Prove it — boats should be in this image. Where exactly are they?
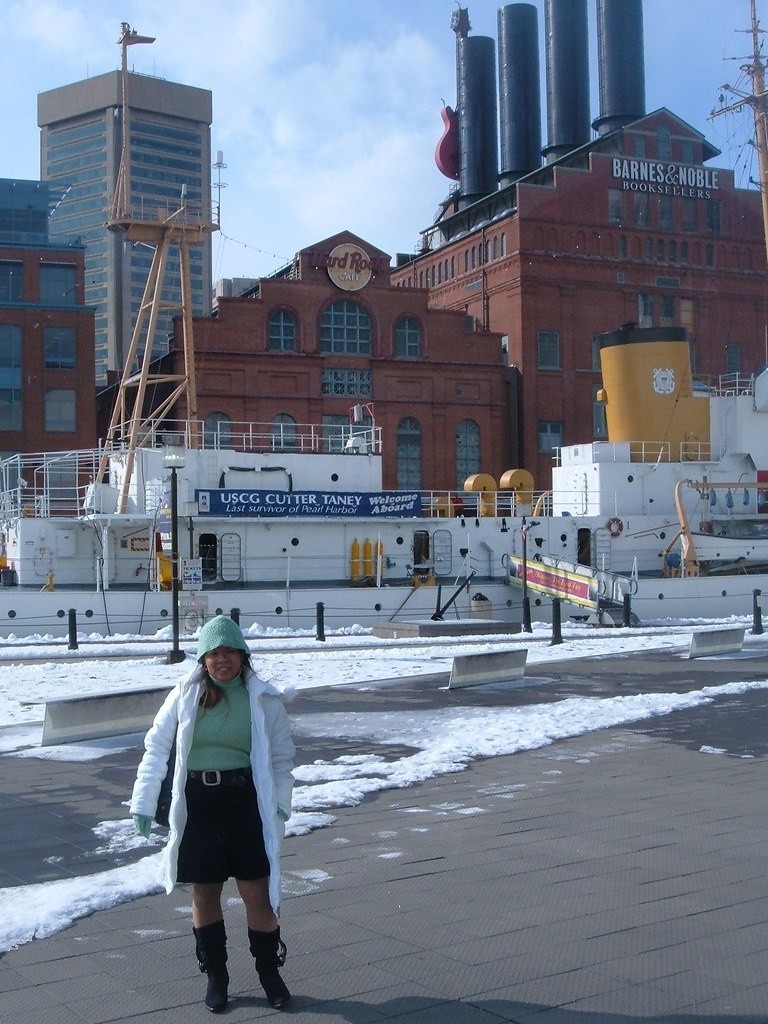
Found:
[689,530,768,567]
[0,19,768,646]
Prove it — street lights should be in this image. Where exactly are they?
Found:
[161,443,186,665]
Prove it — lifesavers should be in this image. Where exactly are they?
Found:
[607,518,623,536]
[184,611,203,631]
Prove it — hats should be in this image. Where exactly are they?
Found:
[196,615,251,664]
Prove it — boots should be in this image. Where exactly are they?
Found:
[247,924,291,1008]
[192,919,230,1012]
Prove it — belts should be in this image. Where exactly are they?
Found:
[188,768,252,785]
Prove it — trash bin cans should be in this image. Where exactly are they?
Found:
[178,595,210,635]
[470,592,493,620]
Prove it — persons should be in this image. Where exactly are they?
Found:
[129,615,295,1012]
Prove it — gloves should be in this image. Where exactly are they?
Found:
[133,814,152,840]
